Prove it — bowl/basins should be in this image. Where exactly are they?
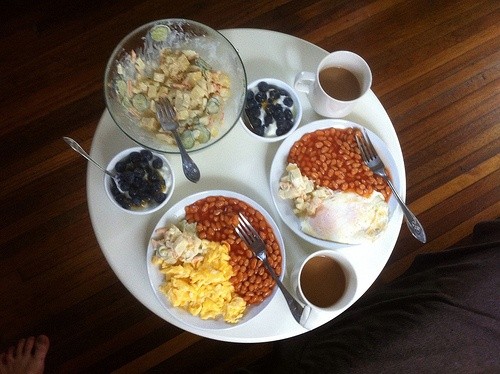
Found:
[240,78,302,142]
[103,17,247,158]
[104,147,176,214]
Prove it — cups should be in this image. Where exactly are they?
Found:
[289,249,358,327]
[293,50,373,119]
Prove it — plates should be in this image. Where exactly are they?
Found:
[269,118,401,250]
[145,188,287,329]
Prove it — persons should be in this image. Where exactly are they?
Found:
[0,335,48,374]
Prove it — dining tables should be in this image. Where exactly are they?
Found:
[86,29,407,344]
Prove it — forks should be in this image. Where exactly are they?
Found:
[233,211,310,327]
[156,96,202,184]
[356,133,428,245]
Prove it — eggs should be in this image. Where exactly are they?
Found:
[297,191,389,246]
